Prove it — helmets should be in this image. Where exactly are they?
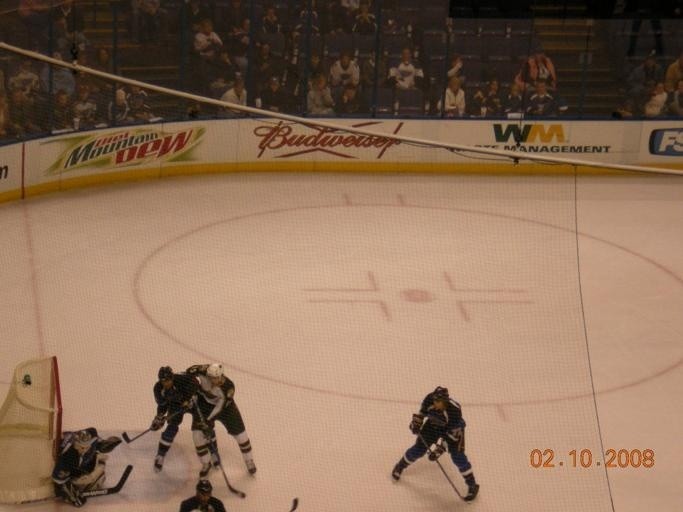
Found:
[205,363,223,377]
[73,430,92,449]
[194,479,212,493]
[430,386,449,402]
[157,366,174,380]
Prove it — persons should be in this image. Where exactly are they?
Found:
[612,52,683,118]
[132,0,559,121]
[392,386,480,500]
[0,0,155,139]
[152,367,220,468]
[52,428,123,506]
[180,479,226,512]
[184,363,256,476]
[625,2,664,57]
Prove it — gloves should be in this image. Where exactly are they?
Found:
[428,444,446,461]
[150,415,165,431]
[409,414,424,435]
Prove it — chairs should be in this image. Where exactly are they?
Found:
[0,0,682,140]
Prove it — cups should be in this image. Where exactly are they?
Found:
[392,100,399,112]
[480,104,487,118]
[423,99,430,112]
[72,118,80,130]
[253,96,261,108]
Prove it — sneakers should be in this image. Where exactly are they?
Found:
[209,451,222,467]
[154,456,163,471]
[463,484,479,501]
[246,460,256,474]
[392,464,402,479]
[198,462,211,477]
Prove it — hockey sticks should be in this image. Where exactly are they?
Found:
[414,427,475,501]
[123,411,183,444]
[195,406,246,498]
[291,497,299,511]
[16,465,132,504]
